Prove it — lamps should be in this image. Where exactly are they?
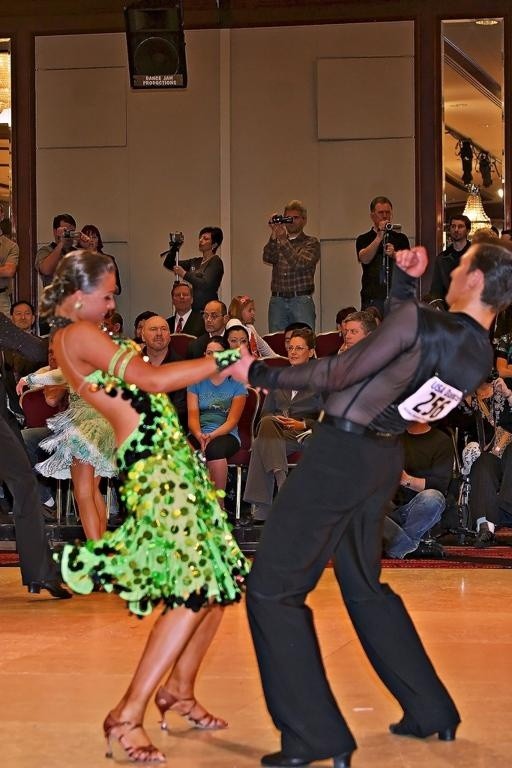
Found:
[459,143,491,241]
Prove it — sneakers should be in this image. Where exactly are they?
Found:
[404,523,494,560]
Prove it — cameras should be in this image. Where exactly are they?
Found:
[169,231,184,243]
[64,229,81,239]
[385,222,402,231]
[272,215,293,224]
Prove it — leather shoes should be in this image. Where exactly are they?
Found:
[261,752,353,768]
[28,580,73,600]
[388,723,458,741]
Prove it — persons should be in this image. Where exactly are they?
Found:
[43,250,242,764]
[3,197,510,597]
[210,242,511,768]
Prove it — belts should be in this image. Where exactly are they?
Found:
[271,289,311,298]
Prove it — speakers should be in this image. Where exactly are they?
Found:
[123,5,188,89]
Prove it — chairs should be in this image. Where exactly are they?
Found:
[20,327,487,528]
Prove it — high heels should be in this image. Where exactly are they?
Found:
[155,684,229,731]
[103,711,167,764]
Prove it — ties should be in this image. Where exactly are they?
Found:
[175,317,184,335]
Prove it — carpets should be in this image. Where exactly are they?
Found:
[0,530,512,570]
